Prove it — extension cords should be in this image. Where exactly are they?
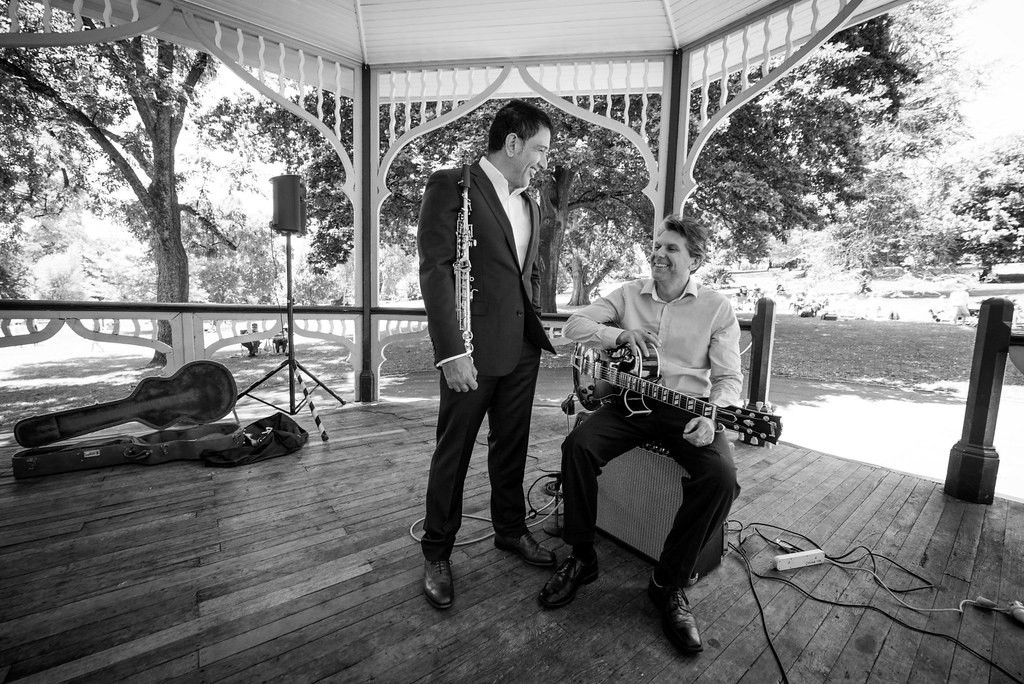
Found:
[773,549,825,570]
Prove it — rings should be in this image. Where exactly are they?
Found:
[702,440,705,445]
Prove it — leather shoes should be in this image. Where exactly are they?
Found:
[494,532,557,566]
[649,570,703,653]
[538,550,599,607]
[423,558,454,608]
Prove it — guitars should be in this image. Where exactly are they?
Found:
[569,321,784,450]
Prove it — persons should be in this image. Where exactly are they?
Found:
[419,100,558,602]
[536,216,744,653]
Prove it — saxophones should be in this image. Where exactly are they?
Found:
[451,149,478,362]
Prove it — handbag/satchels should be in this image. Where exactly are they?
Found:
[200,412,309,468]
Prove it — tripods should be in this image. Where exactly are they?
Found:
[235,233,347,442]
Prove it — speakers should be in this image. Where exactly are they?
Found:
[268,175,306,235]
[573,411,724,586]
